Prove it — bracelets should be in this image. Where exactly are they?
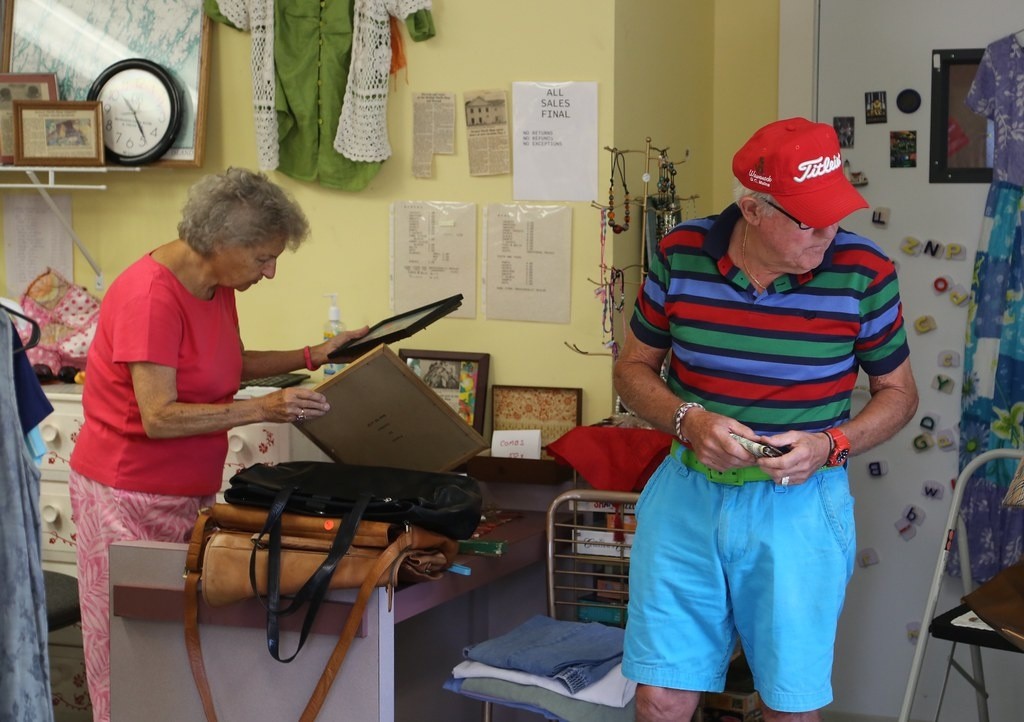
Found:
[820,431,832,467]
[304,346,320,371]
[672,402,705,443]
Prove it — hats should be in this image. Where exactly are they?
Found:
[733,117,870,229]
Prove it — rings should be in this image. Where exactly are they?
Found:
[782,476,789,485]
[297,408,306,421]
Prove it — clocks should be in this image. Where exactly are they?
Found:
[87,59,182,165]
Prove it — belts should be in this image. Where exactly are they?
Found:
[670,440,773,485]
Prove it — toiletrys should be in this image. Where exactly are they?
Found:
[319,293,347,375]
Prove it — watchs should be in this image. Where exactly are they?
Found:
[825,428,851,467]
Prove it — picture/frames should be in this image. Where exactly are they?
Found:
[329,292,464,358]
[294,343,489,472]
[0,0,213,167]
[0,71,60,165]
[490,384,582,459]
[397,349,490,474]
[13,98,105,166]
[928,46,996,183]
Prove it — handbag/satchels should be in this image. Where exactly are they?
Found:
[184,457,487,722]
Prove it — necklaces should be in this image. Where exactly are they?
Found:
[742,223,766,289]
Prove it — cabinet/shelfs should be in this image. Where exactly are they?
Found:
[25,384,86,564]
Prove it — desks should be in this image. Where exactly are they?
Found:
[107,508,583,722]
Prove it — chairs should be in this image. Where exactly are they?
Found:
[482,489,704,722]
[900,449,1024,722]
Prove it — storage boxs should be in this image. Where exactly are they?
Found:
[572,528,636,557]
[597,579,629,598]
[568,501,636,514]
[605,513,636,530]
[704,687,762,722]
[576,593,628,624]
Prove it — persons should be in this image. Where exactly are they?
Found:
[613,117,919,722]
[67,168,369,722]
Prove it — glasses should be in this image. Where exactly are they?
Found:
[761,197,812,230]
